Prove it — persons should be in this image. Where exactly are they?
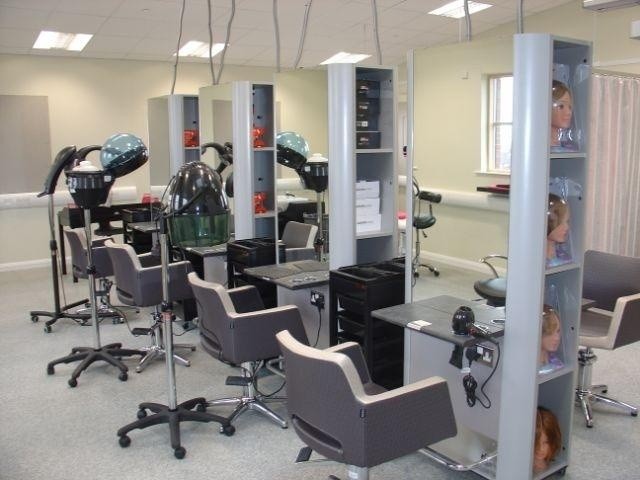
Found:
[551,80,572,146]
[541,303,562,367]
[547,192,571,260]
[533,407,562,472]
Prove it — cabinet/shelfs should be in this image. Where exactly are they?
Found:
[496,32,594,480]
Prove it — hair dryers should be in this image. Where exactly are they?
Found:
[447,306,499,369]
